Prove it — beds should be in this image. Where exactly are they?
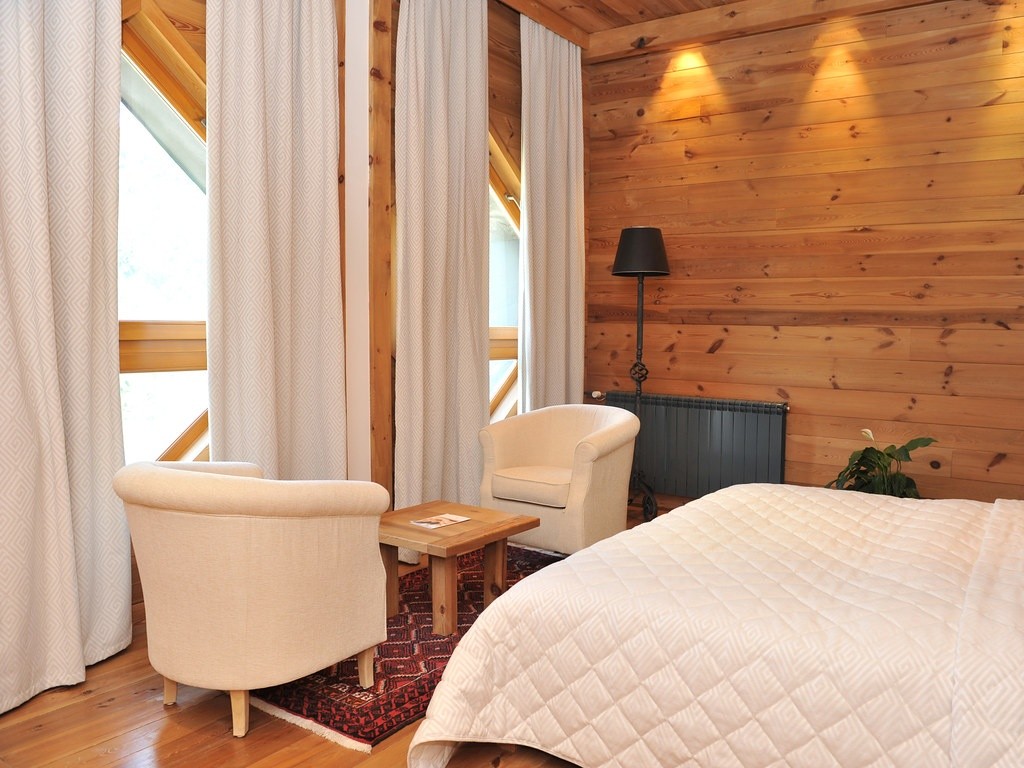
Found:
[406,483,1024,768]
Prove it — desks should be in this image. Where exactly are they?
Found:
[380,500,540,637]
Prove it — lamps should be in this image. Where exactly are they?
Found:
[609,227,670,514]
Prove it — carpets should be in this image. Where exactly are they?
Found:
[222,543,566,754]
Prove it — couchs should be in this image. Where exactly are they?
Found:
[113,459,391,739]
[479,403,642,558]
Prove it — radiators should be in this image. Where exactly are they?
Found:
[592,390,792,499]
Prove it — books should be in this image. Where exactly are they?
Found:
[410,513,471,529]
